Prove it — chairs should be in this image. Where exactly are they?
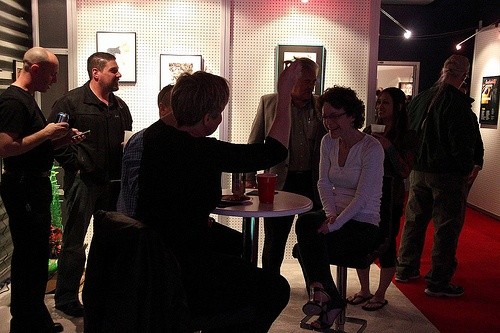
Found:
[92,209,198,333]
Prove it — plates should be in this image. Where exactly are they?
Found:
[221,194,253,204]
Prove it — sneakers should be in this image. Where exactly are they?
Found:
[425,284,465,297]
[395,270,421,282]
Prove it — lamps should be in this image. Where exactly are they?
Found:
[456,23,494,50]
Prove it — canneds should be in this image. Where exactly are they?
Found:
[55,112,69,123]
[232,173,246,195]
[246,172,257,188]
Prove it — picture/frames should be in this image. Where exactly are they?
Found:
[95,31,136,84]
[159,53,203,91]
[277,44,326,97]
[12,60,23,82]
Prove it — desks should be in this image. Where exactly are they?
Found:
[212,188,313,270]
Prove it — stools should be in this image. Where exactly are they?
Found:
[293,242,380,332]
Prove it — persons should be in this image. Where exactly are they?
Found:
[348,87,418,309]
[295,86,385,329]
[244,58,321,273]
[47,51,132,318]
[0,47,86,333]
[394,55,485,296]
[116,60,302,333]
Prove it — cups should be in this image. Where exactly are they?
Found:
[231,172,246,201]
[257,173,278,206]
[371,123,385,138]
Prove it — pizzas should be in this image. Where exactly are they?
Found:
[221,195,241,200]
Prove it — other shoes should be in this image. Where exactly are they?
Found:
[55,302,84,317]
[53,322,63,331]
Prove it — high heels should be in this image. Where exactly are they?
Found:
[311,303,346,328]
[302,287,332,316]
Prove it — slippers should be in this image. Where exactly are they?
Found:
[346,293,373,304]
[362,298,388,311]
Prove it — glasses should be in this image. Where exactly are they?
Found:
[319,112,345,121]
